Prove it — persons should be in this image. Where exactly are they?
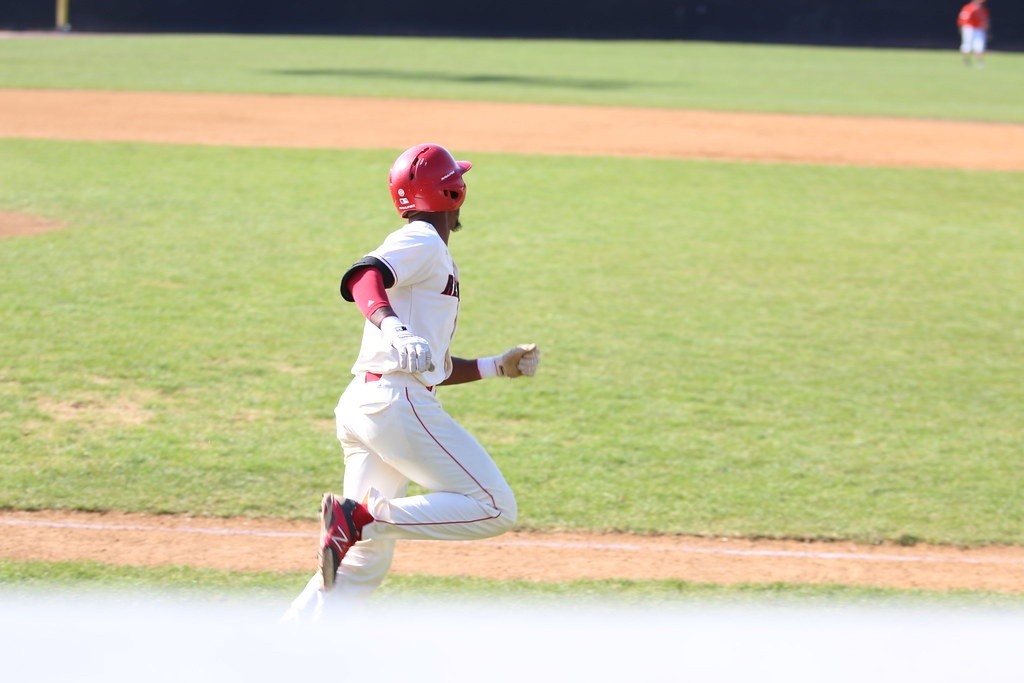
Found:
[957,0,990,63]
[277,143,539,618]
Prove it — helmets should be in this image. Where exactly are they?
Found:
[388,144,473,219]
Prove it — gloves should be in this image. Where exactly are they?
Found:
[477,343,539,380]
[380,315,436,374]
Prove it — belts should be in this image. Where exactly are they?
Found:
[365,372,433,393]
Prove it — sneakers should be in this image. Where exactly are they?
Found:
[320,492,363,593]
